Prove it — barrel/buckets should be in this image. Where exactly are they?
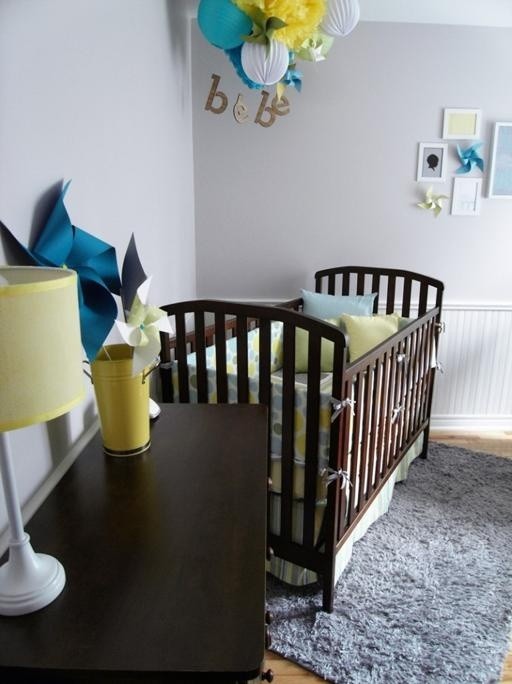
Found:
[82,343,160,457]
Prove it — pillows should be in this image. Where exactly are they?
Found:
[288,287,402,375]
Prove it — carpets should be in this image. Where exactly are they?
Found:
[267,439,512,683]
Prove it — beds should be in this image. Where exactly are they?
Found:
[156,265,445,614]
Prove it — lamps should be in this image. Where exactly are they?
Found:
[0,266,98,617]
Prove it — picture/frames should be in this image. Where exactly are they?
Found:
[414,106,511,217]
